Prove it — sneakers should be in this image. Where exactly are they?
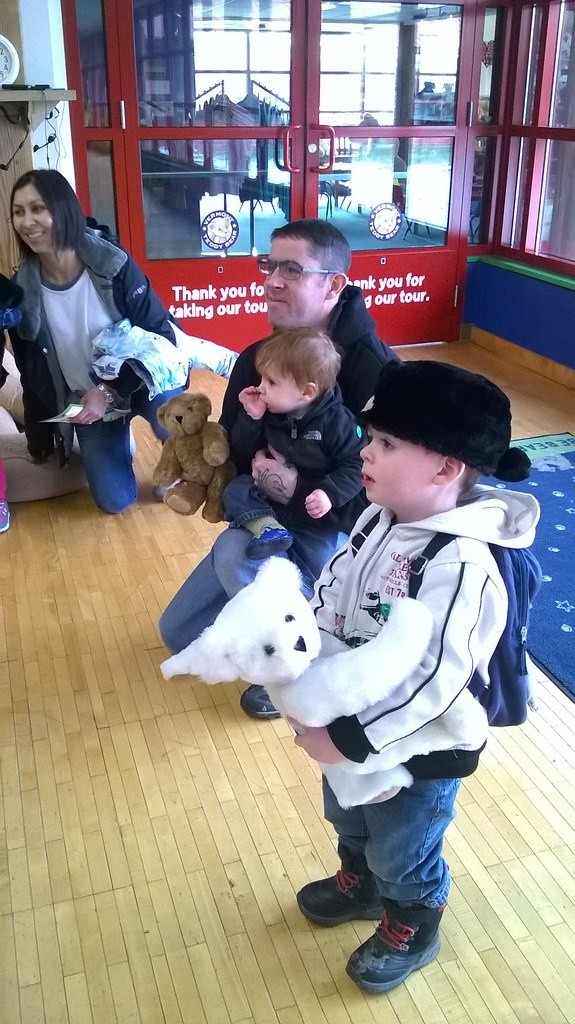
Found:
[0,499,10,533]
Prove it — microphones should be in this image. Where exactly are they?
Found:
[0,140,26,171]
[34,135,57,152]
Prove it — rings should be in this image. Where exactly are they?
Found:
[88,421,92,424]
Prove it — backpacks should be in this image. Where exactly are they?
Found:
[349,507,542,728]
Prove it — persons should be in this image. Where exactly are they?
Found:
[0,169,184,533]
[224,328,367,582]
[291,360,539,994]
[160,218,400,721]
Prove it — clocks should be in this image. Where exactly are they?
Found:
[0,34,20,91]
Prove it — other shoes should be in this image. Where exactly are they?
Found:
[128,421,136,461]
[152,476,181,501]
[245,524,294,560]
[240,684,284,719]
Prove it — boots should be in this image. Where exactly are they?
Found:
[297,843,384,926]
[346,896,447,993]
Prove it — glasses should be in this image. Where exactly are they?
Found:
[256,257,340,280]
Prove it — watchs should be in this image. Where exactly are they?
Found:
[97,384,115,404]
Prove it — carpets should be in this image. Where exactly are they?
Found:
[476,432,575,700]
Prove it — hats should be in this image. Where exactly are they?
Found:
[356,359,531,482]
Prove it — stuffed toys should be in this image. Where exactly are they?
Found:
[153,393,235,523]
[163,556,486,809]
